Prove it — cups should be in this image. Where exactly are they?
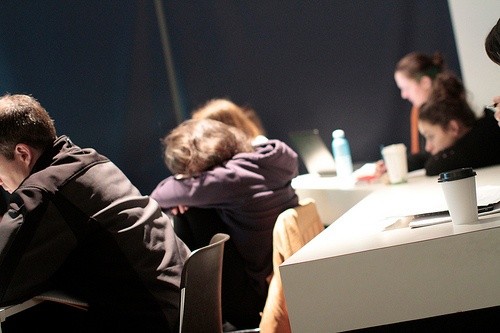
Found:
[438,167,479,226]
[381,143,408,184]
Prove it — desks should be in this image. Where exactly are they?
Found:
[279,163,500,333]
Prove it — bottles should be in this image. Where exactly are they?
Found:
[331,130,354,178]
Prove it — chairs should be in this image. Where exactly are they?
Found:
[178,233,230,332]
[258,201,326,333]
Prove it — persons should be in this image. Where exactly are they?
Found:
[150,98,299,333]
[0,94,191,333]
[376,18,500,176]
